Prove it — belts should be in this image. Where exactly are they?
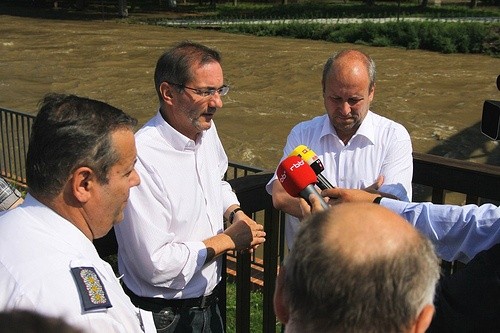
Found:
[125,286,221,310]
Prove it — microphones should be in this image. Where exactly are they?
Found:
[276,154,330,210]
[288,144,337,198]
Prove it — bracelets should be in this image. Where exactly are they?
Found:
[229,208,242,225]
[373,197,382,204]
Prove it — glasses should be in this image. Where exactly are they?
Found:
[163,79,232,98]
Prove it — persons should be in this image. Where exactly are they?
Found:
[0,92,158,333]
[320,188,500,264]
[273,201,440,333]
[266,49,413,254]
[113,42,267,333]
